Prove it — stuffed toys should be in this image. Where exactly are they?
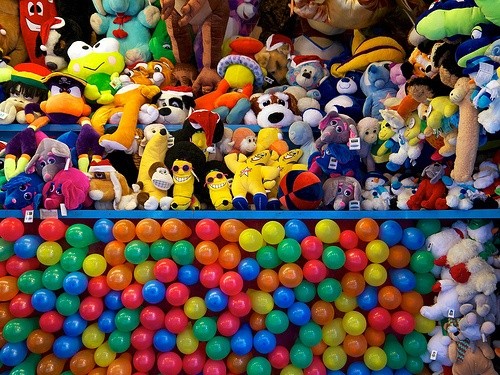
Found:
[420,220,500,375]
[0,0,500,210]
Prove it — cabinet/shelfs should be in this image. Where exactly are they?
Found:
[0,123,500,220]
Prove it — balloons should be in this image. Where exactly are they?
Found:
[0,216,441,375]
